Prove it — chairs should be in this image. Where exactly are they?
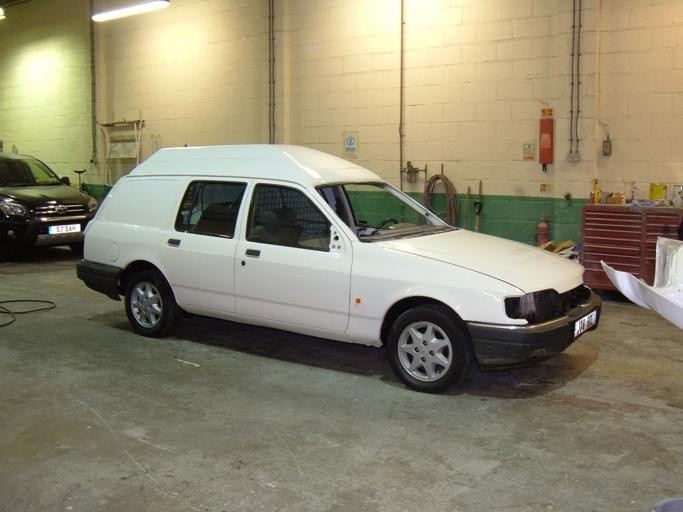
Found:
[255,208,303,244]
[191,204,234,237]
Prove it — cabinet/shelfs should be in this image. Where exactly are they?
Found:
[580,205,682,291]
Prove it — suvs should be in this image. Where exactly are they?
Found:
[0,152,100,258]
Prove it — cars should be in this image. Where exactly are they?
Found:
[73,143,601,392]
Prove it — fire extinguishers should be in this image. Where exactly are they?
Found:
[537,217,548,246]
